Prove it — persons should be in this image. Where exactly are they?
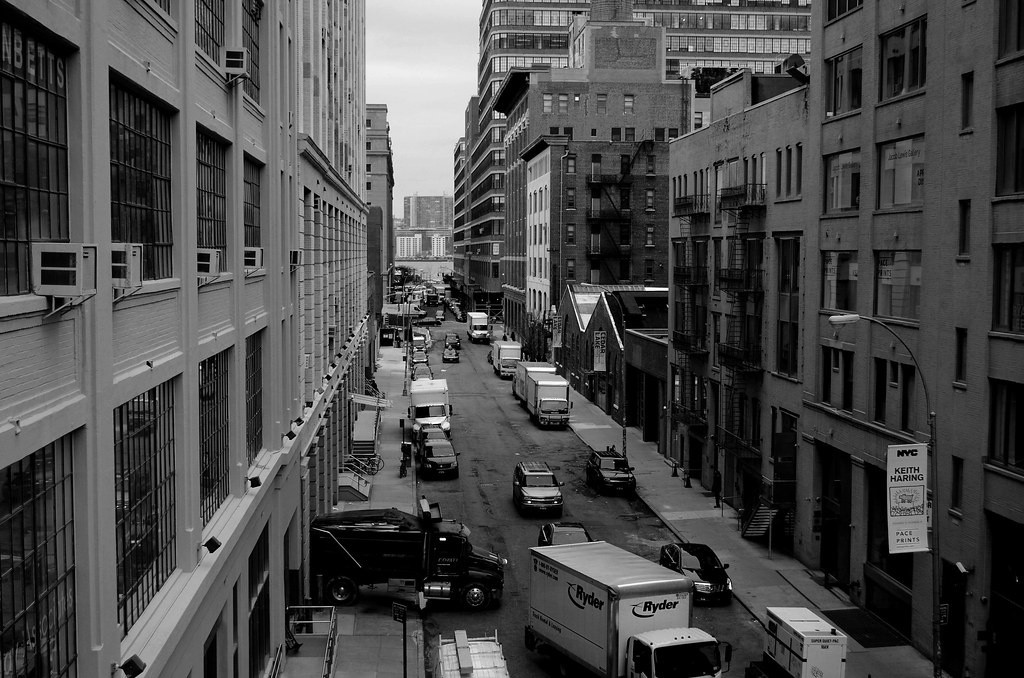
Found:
[502,334,507,341]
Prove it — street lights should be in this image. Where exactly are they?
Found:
[831,313,943,678]
[402,268,424,353]
[580,283,626,457]
[403,279,440,395]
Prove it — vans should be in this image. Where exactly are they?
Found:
[427,294,439,307]
[381,303,427,318]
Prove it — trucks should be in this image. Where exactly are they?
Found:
[494,340,522,378]
[523,541,733,678]
[516,361,558,409]
[467,312,491,344]
[309,502,507,609]
[407,378,453,440]
[433,285,446,300]
[525,372,573,428]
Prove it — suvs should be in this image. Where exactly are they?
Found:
[513,460,565,515]
[587,449,637,494]
[417,424,453,456]
[538,521,591,547]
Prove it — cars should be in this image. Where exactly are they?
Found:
[443,333,462,349]
[411,336,434,380]
[421,439,460,480]
[436,310,445,321]
[487,350,494,364]
[412,317,442,327]
[442,298,467,322]
[660,543,733,603]
[441,345,460,363]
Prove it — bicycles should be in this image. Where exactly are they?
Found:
[346,454,384,475]
[399,459,408,478]
